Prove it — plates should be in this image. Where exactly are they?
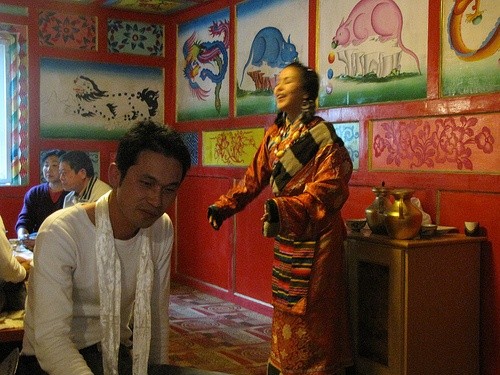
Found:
[435,225,456,234]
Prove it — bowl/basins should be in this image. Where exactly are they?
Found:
[345,218,367,233]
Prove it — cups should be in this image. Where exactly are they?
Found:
[419,224,437,239]
[463,222,479,237]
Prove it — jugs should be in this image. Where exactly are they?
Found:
[383,190,422,240]
[365,186,393,235]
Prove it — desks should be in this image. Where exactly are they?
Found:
[9,239,35,293]
[342,223,488,375]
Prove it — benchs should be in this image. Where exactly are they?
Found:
[0,307,25,343]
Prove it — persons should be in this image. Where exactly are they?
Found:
[0,215,31,362]
[15,149,114,252]
[207,62,353,375]
[18,119,226,375]
